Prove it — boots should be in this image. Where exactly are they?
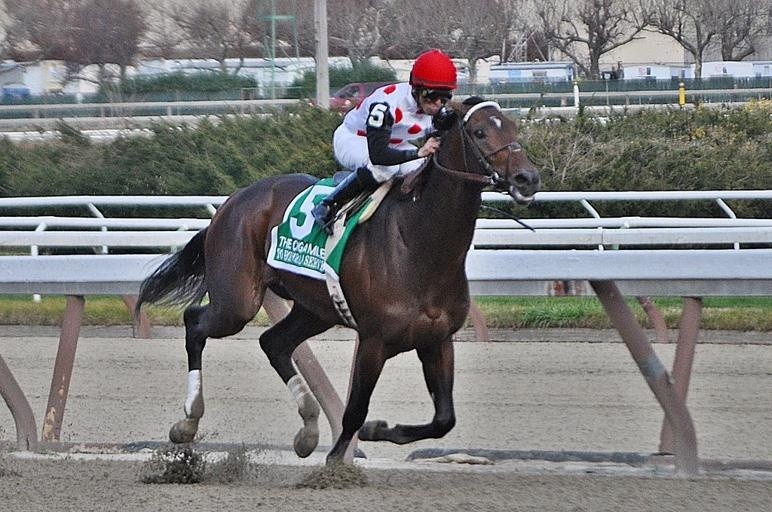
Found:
[309,165,379,236]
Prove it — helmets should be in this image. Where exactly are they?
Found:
[408,48,459,91]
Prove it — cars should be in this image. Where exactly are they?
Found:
[308,80,393,113]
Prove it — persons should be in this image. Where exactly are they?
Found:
[309,47,459,236]
[608,64,619,79]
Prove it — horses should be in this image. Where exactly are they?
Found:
[131,96,543,469]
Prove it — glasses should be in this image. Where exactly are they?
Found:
[420,87,453,102]
